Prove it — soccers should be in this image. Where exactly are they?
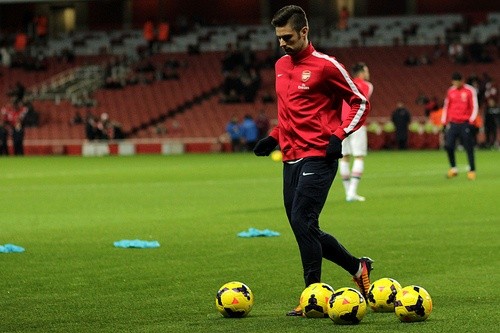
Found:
[216,282,254,319]
[394,285,432,322]
[271,150,282,161]
[328,288,366,325]
[299,283,334,318]
[368,279,402,312]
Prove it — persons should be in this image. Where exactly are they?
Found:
[336,61,373,202]
[441,72,481,181]
[254,5,375,317]
[0,19,499,157]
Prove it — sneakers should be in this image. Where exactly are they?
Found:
[352,257,374,303]
[286,303,302,316]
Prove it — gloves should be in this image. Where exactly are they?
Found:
[253,136,278,156]
[326,135,343,163]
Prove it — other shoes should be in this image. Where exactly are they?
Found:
[447,168,459,177]
[346,195,365,201]
[468,171,475,180]
